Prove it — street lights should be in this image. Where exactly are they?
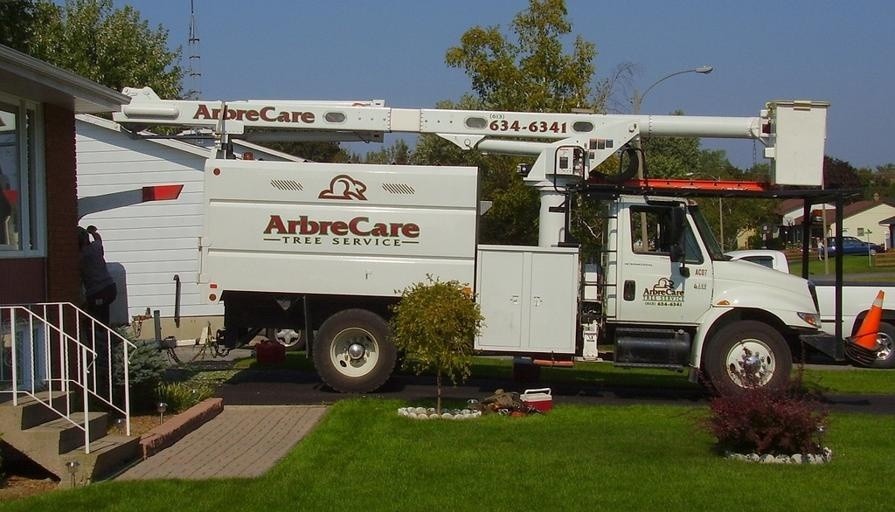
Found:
[632,66,714,253]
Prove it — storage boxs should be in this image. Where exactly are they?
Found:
[520,387,553,410]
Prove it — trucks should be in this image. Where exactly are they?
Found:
[110,87,830,405]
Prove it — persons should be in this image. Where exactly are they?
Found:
[78,226,116,332]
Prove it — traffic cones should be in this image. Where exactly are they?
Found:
[845,289,885,365]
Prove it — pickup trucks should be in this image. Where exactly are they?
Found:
[721,249,895,368]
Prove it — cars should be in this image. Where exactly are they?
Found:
[819,237,877,258]
